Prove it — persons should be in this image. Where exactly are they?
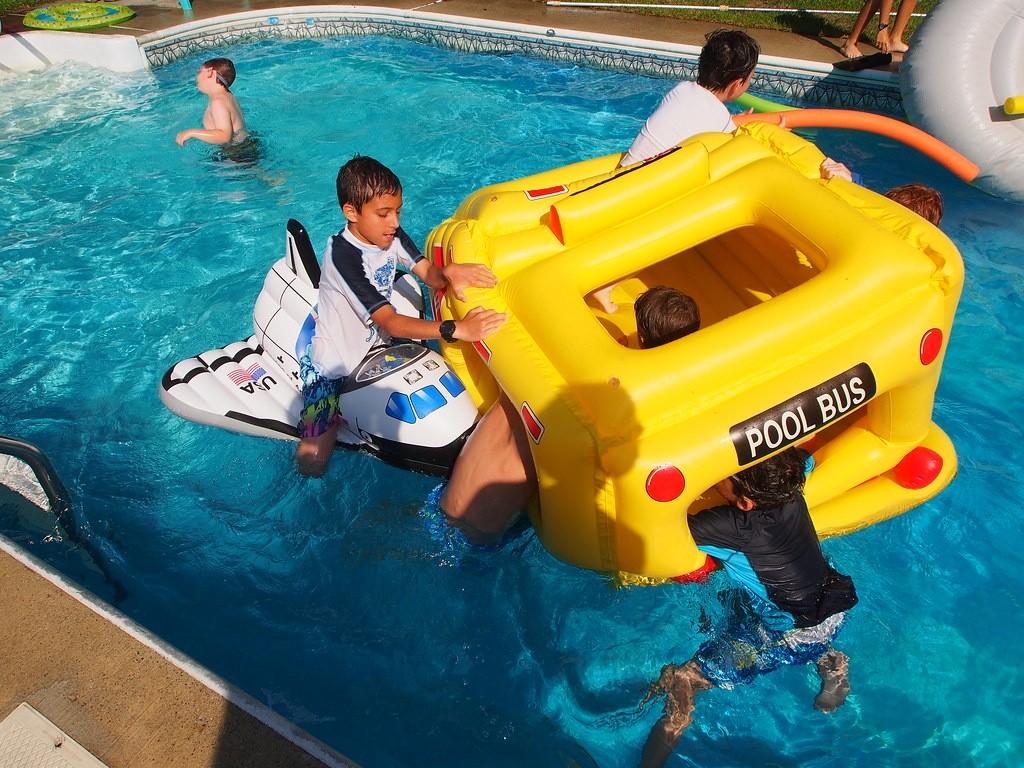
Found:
[633,286,701,350]
[840,0,918,58]
[687,446,845,608]
[591,26,791,313]
[176,58,246,146]
[820,157,943,228]
[299,153,507,459]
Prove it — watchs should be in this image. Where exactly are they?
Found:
[878,23,890,30]
[439,320,457,343]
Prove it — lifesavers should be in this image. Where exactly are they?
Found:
[730,91,982,183]
